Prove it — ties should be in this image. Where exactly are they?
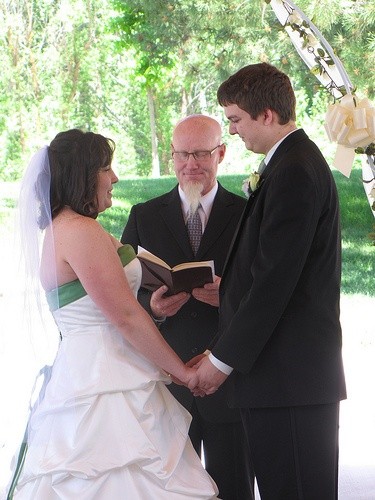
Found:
[188,211,203,253]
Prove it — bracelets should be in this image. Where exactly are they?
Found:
[169,373,171,377]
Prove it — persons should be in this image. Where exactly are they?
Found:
[119,115,250,499]
[171,63,347,500]
[11,128,219,500]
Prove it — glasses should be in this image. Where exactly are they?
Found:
[171,143,221,162]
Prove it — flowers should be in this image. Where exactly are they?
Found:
[241,171,261,198]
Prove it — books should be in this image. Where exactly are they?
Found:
[135,244,215,298]
